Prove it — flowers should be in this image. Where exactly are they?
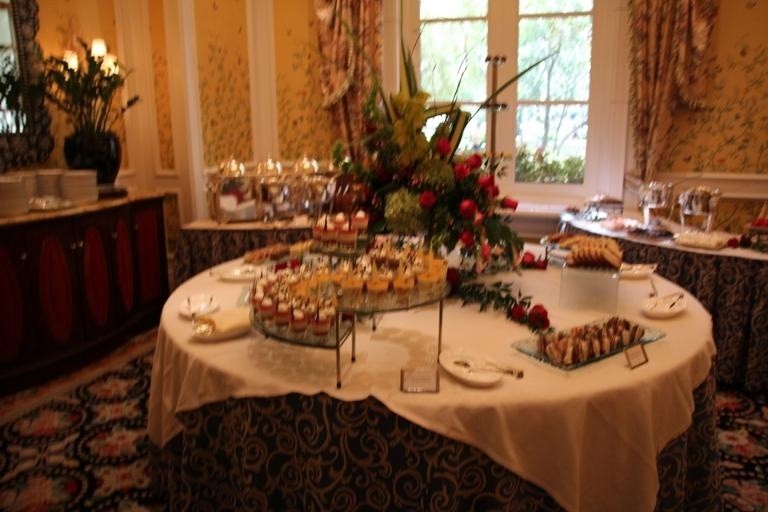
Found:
[333,30,554,334]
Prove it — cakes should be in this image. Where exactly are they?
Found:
[249,209,449,334]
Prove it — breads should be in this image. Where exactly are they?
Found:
[538,316,645,366]
[565,237,624,270]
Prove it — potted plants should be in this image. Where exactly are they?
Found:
[37,40,140,187]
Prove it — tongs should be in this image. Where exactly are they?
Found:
[186,296,213,319]
[453,357,524,381]
[650,292,684,311]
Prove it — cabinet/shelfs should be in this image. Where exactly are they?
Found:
[0,189,169,397]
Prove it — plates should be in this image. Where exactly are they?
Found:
[437,349,503,388]
[177,294,218,319]
[618,263,651,279]
[639,295,688,319]
[0,166,100,218]
[220,263,262,282]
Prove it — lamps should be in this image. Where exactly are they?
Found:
[59,36,120,81]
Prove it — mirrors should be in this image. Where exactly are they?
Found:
[0,0,56,176]
